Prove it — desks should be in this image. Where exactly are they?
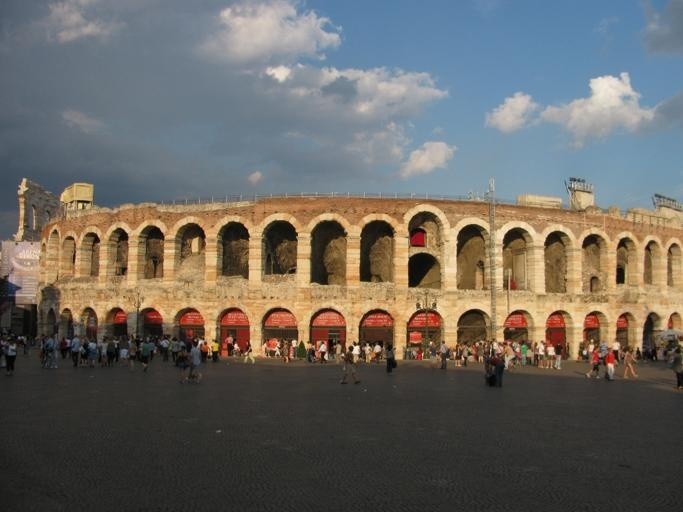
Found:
[127,289,144,339]
[416,289,437,359]
